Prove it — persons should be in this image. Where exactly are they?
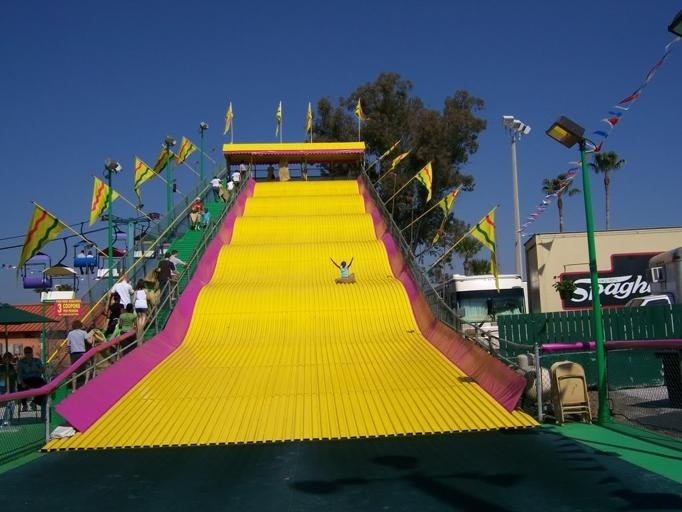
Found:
[329,255,356,284]
[227,179,234,194]
[232,169,242,190]
[1,351,19,426]
[15,346,47,412]
[65,249,190,387]
[192,197,204,212]
[189,207,211,231]
[323,166,331,176]
[239,161,247,180]
[267,163,275,180]
[209,176,223,202]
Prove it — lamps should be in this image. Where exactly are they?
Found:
[553,363,593,424]
[16,382,38,421]
[550,359,587,422]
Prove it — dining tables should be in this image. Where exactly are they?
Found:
[552,275,578,301]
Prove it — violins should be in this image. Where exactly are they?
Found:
[38,175,542,453]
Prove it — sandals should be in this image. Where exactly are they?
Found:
[424,273,529,350]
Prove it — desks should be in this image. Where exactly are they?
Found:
[502,114,612,425]
[103,120,209,288]
